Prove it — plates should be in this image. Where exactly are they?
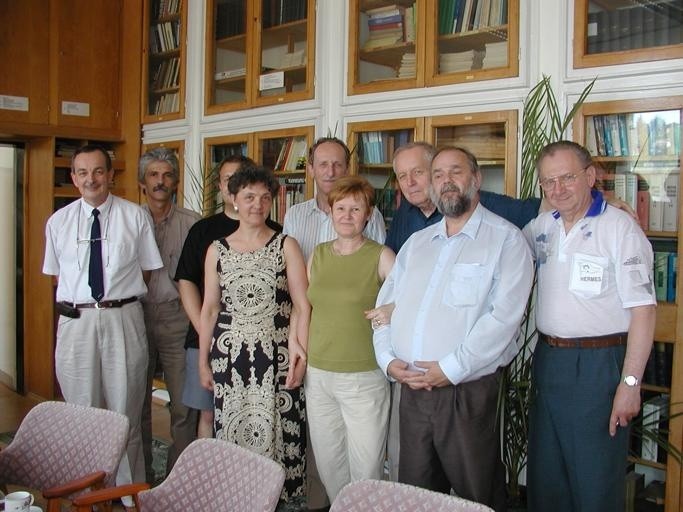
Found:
[31,506,44,512]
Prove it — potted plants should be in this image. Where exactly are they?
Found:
[485,69,596,512]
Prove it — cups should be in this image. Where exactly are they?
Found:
[3,491,34,512]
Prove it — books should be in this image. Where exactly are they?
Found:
[59,148,117,188]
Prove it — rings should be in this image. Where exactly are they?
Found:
[376,321,380,325]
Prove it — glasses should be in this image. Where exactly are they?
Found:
[538,163,592,189]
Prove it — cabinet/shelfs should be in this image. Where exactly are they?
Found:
[20,134,130,404]
[570,0,682,71]
[137,0,189,124]
[343,1,523,98]
[199,1,317,117]
[138,139,185,395]
[571,93,682,510]
[199,124,317,231]
[344,106,521,204]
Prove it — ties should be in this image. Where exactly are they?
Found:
[85,209,105,302]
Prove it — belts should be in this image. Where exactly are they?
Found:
[535,328,632,352]
[60,294,141,311]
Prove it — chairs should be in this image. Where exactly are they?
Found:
[69,436,287,512]
[0,398,132,511]
[325,477,494,512]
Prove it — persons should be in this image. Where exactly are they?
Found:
[197,166,312,512]
[384,141,639,483]
[371,146,535,511]
[42,143,165,512]
[282,137,387,512]
[172,154,284,438]
[135,146,203,486]
[286,173,396,506]
[521,141,657,511]
